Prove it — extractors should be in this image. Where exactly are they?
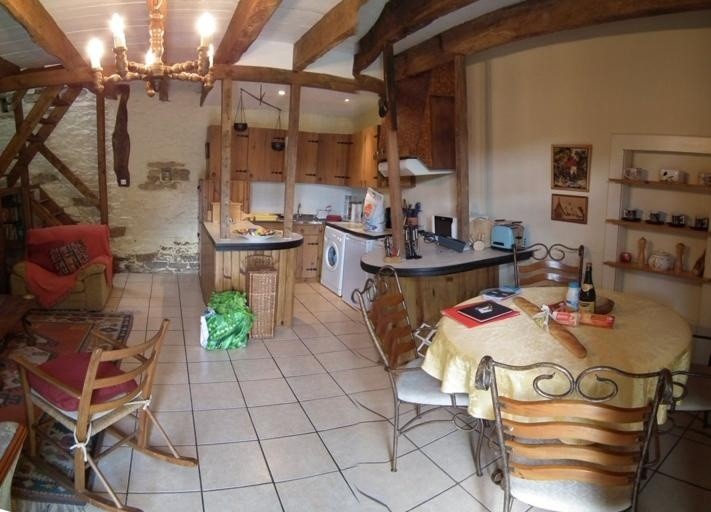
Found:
[377,157,455,176]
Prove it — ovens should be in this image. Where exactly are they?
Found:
[342,235,371,313]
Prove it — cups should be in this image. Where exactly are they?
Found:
[673,216,685,224]
[624,209,638,218]
[697,171,711,185]
[624,167,643,181]
[650,211,665,222]
[695,216,710,229]
[514,236,524,251]
[620,252,633,262]
[408,217,418,227]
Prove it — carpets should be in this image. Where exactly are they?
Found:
[0,310,134,510]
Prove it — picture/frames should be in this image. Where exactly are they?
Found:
[550,142,592,192]
[550,193,591,226]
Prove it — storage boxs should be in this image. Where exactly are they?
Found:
[209,201,243,225]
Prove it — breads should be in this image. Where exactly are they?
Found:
[513,297,587,360]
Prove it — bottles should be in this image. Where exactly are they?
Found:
[674,243,686,274]
[638,236,646,265]
[579,261,597,316]
[344,194,352,220]
[649,248,673,272]
[566,281,579,311]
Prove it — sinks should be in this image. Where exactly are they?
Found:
[288,215,316,221]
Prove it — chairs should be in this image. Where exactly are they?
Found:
[638,334,710,478]
[474,352,683,510]
[5,317,198,512]
[350,264,490,477]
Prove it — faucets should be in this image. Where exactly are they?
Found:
[297,203,301,216]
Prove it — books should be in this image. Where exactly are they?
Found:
[457,300,513,323]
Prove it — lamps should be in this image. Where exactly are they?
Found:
[86,0,217,99]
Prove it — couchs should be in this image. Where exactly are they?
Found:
[11,221,114,310]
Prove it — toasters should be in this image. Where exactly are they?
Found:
[492,222,526,249]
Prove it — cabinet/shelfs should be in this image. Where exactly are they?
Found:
[284,132,319,184]
[299,223,323,282]
[348,121,417,189]
[320,134,348,187]
[247,128,283,182]
[206,125,249,181]
[601,177,711,286]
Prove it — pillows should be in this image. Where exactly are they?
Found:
[47,239,89,274]
[26,239,62,272]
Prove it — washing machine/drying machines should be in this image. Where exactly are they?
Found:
[342,228,393,312]
[320,221,363,297]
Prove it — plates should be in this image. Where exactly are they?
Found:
[596,295,615,316]
[645,220,665,224]
[228,232,281,241]
[668,222,686,227]
[689,226,708,231]
[622,217,641,222]
